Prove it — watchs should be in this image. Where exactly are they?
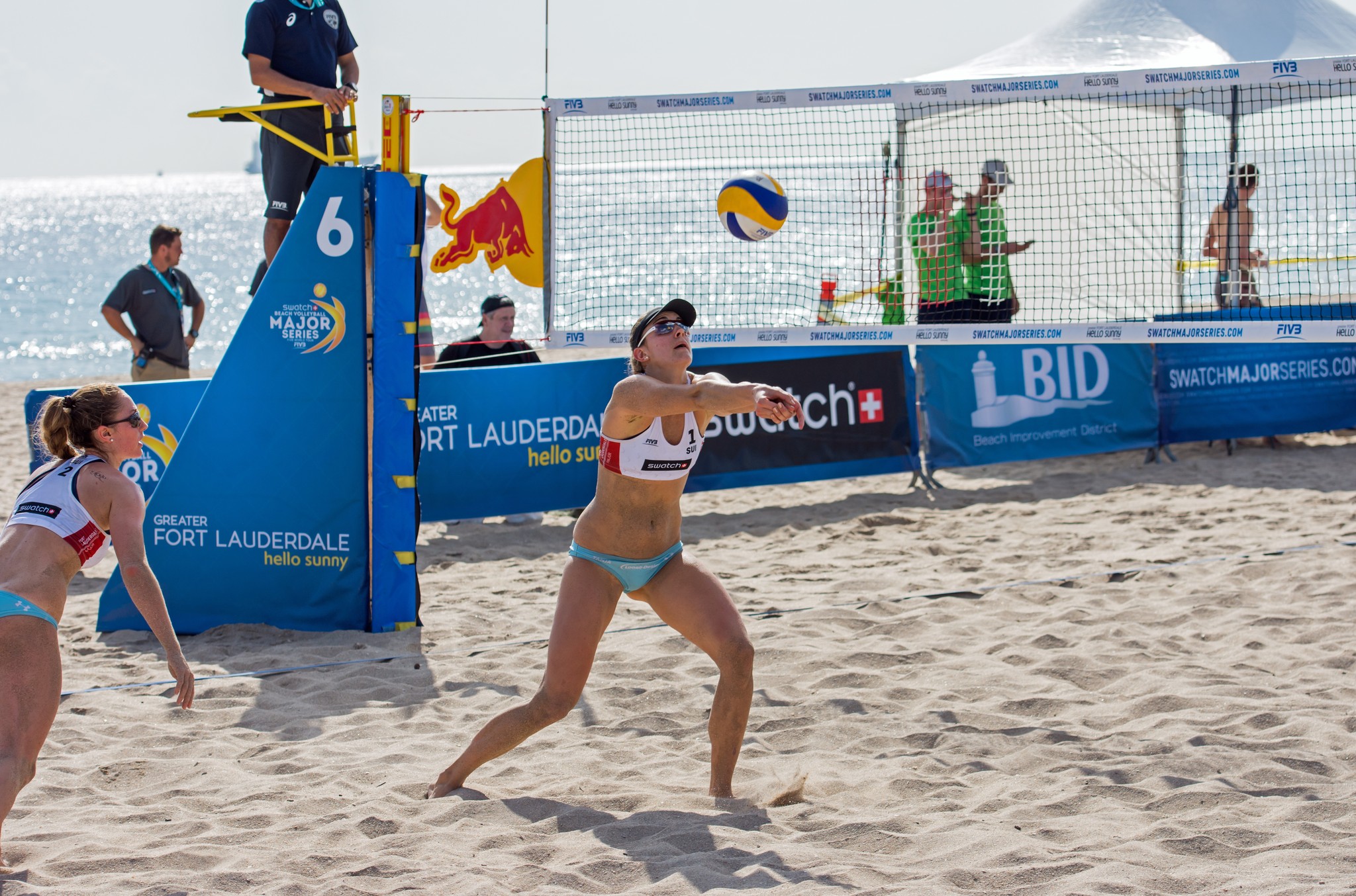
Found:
[343,81,358,92]
[189,329,199,338]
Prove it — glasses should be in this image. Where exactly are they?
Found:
[83,411,141,434]
[637,320,690,348]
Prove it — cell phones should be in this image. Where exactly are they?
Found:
[1025,240,1035,245]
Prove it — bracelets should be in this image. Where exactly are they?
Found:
[996,245,1002,257]
[967,211,977,217]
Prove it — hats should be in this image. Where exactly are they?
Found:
[925,171,959,188]
[477,294,514,327]
[632,299,697,356]
[981,159,1013,187]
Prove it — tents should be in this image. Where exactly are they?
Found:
[879,0,1356,315]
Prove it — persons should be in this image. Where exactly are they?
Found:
[0,381,195,867]
[909,171,981,324]
[955,159,1035,324]
[432,294,541,526]
[875,270,906,325]
[426,298,753,799]
[242,0,360,272]
[1203,164,1267,307]
[101,225,205,382]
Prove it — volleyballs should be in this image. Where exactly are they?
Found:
[717,171,788,242]
